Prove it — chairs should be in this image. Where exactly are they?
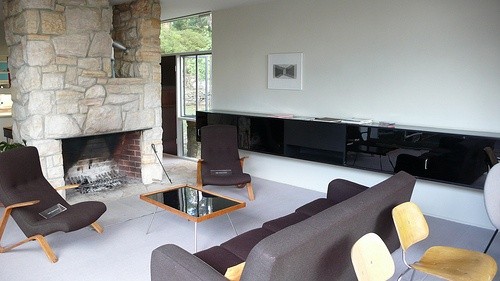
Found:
[0,147,106,265]
[196,124,254,203]
[391,202,498,281]
[351,233,395,281]
[482,147,498,174]
[483,163,500,254]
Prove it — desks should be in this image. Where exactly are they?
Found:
[345,139,399,171]
[140,185,245,254]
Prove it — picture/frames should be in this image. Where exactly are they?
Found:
[266,52,302,91]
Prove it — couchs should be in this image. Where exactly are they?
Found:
[151,168,418,281]
[394,132,495,180]
